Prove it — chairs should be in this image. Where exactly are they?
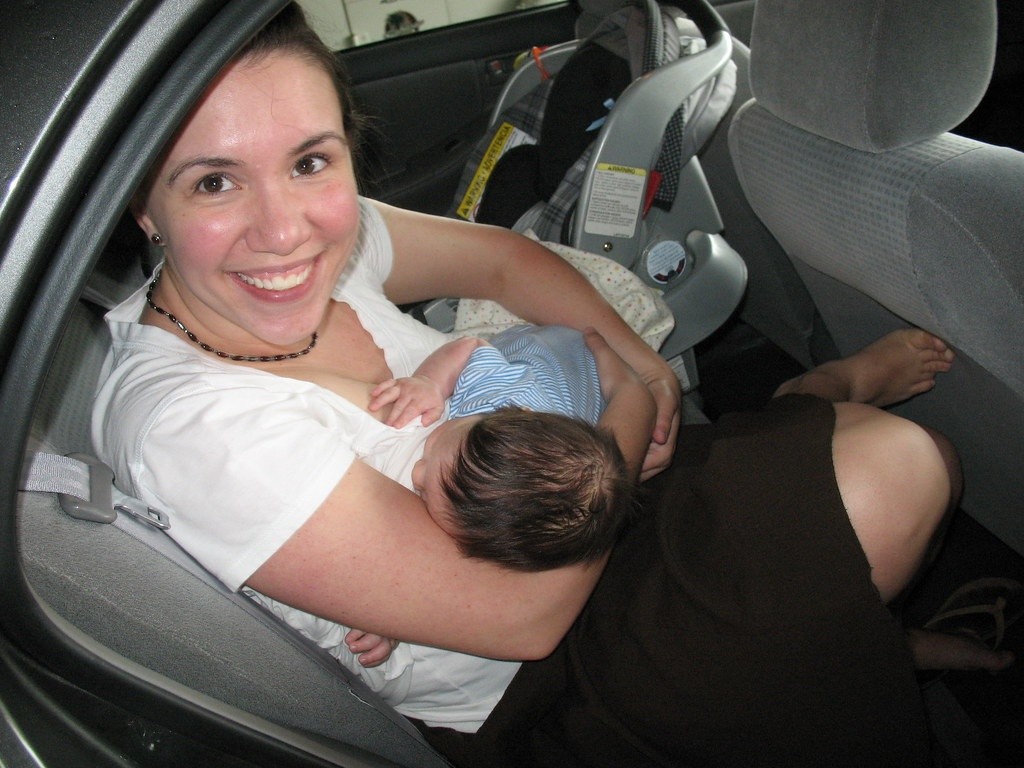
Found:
[727,0,1024,556]
[474,6,707,229]
[18,266,450,768]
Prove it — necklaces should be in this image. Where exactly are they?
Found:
[146,269,318,362]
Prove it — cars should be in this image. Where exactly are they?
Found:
[0,1,1024,768]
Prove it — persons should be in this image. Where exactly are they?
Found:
[344,226,675,669]
[90,6,1013,768]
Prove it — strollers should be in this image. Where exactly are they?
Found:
[396,1,747,404]
